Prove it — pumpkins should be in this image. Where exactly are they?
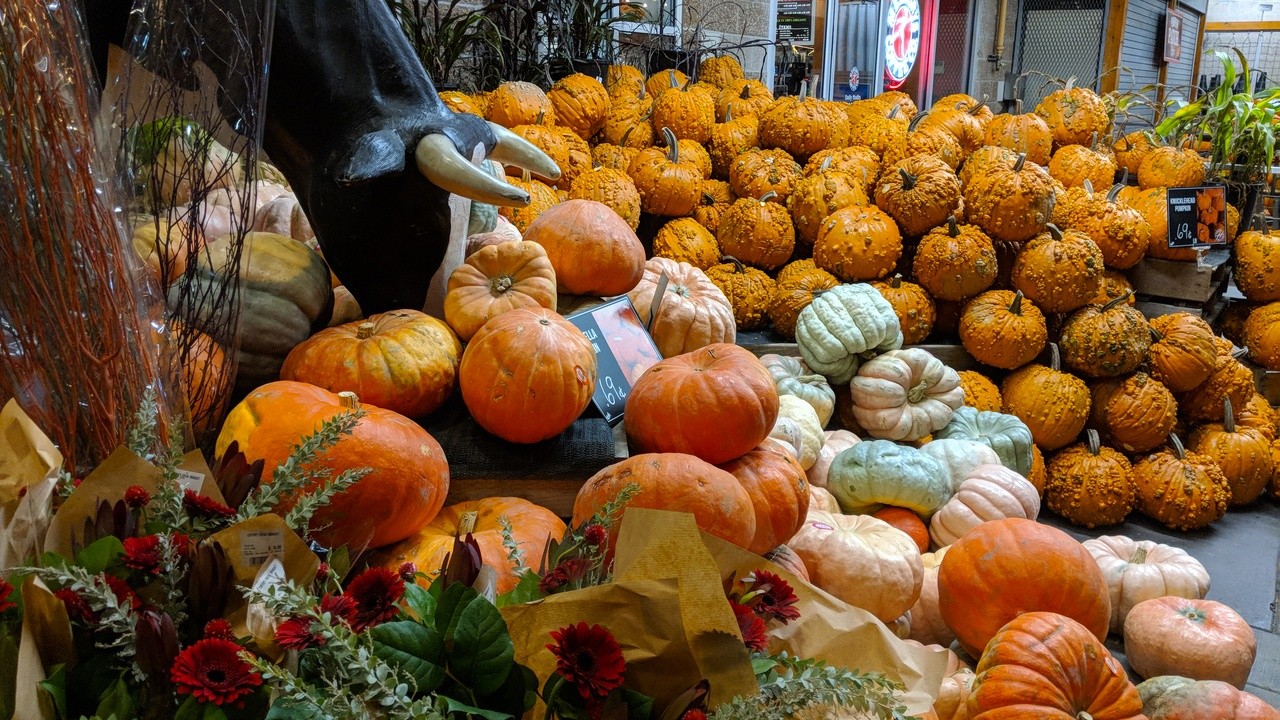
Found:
[125,54,1279,719]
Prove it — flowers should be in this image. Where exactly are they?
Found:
[0,368,931,720]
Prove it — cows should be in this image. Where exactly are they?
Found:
[80,1,563,319]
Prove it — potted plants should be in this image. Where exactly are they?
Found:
[1014,41,1280,234]
[384,0,798,96]
